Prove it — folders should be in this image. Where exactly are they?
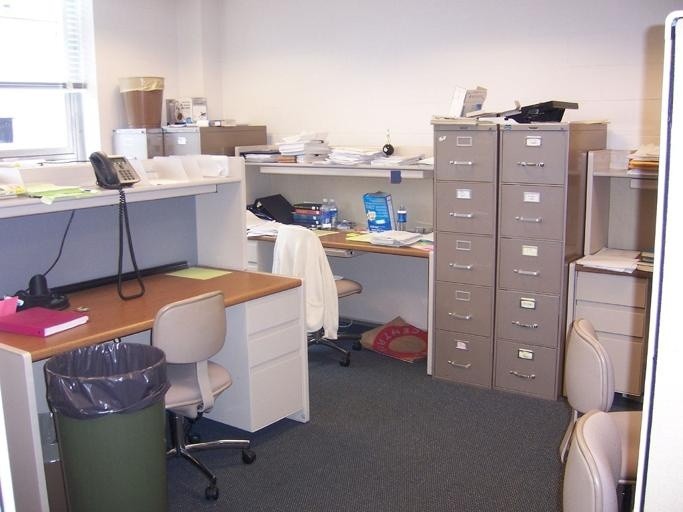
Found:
[254,193,294,225]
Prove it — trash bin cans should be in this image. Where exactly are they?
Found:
[43,341,168,512]
[118,76,164,129]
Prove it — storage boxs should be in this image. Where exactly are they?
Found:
[166,125,268,157]
[111,129,166,160]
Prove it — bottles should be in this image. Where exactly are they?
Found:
[396,205,407,231]
[328,198,337,229]
[319,198,330,225]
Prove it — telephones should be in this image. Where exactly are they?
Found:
[89,152,141,188]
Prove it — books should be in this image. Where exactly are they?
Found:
[626,144,662,178]
[572,246,655,274]
[289,198,323,225]
[239,132,435,168]
[0,303,89,339]
[433,85,581,124]
[351,228,434,250]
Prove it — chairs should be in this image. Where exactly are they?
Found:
[151,291,257,500]
[560,316,641,512]
[561,409,624,512]
[271,225,363,366]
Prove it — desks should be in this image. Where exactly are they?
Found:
[1,154,311,512]
[234,156,434,375]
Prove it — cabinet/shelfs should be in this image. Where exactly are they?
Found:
[432,121,498,394]
[494,119,608,404]
[562,147,662,406]
